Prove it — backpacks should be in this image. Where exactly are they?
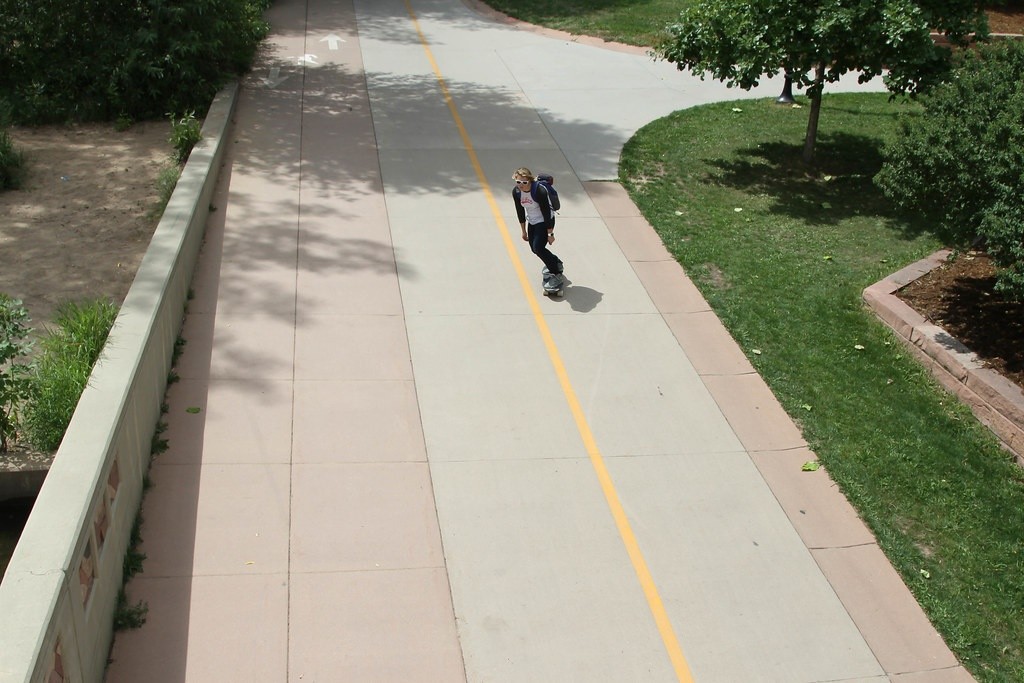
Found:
[516,173,560,211]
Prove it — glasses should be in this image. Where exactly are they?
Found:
[515,179,530,185]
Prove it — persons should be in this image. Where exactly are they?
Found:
[512,168,563,289]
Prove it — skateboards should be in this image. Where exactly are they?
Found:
[542,261,564,297]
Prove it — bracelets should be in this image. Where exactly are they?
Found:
[548,233,554,236]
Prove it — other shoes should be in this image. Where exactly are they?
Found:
[544,274,563,288]
[542,268,551,274]
